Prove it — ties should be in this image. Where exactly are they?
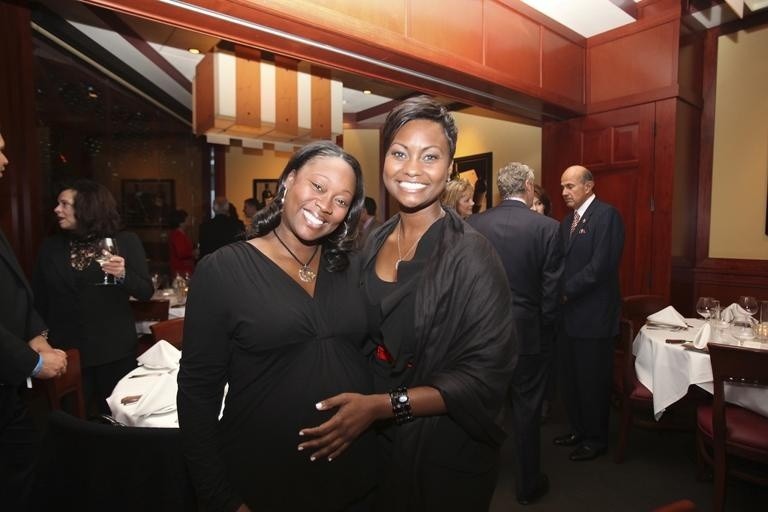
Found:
[569,212,579,235]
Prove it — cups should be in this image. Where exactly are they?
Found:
[177,279,183,303]
[696,297,715,327]
[150,272,161,297]
[737,296,759,328]
[730,316,757,346]
[760,301,768,349]
[94,238,119,285]
[710,300,720,344]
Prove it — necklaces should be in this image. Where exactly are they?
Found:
[273,228,319,283]
[395,214,442,272]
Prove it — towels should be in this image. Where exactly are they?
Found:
[694,322,741,349]
[129,373,179,419]
[648,306,692,328]
[722,304,759,325]
[168,307,185,316]
[136,340,181,371]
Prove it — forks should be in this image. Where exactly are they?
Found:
[132,368,177,377]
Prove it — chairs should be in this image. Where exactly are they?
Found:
[697,343,768,507]
[620,292,662,324]
[149,318,184,350]
[614,319,654,462]
[128,298,170,336]
[43,348,87,420]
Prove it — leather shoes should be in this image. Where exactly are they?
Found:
[570,441,602,463]
[554,435,582,448]
[517,472,549,505]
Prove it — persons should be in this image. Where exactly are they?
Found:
[167,208,200,286]
[262,190,274,206]
[242,198,265,220]
[297,94,520,512]
[553,165,624,463]
[0,134,70,512]
[530,182,552,216]
[358,196,384,249]
[439,177,476,220]
[176,140,375,512]
[199,196,244,258]
[33,180,156,423]
[466,161,566,505]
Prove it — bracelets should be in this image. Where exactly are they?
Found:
[388,386,414,426]
[31,354,44,377]
